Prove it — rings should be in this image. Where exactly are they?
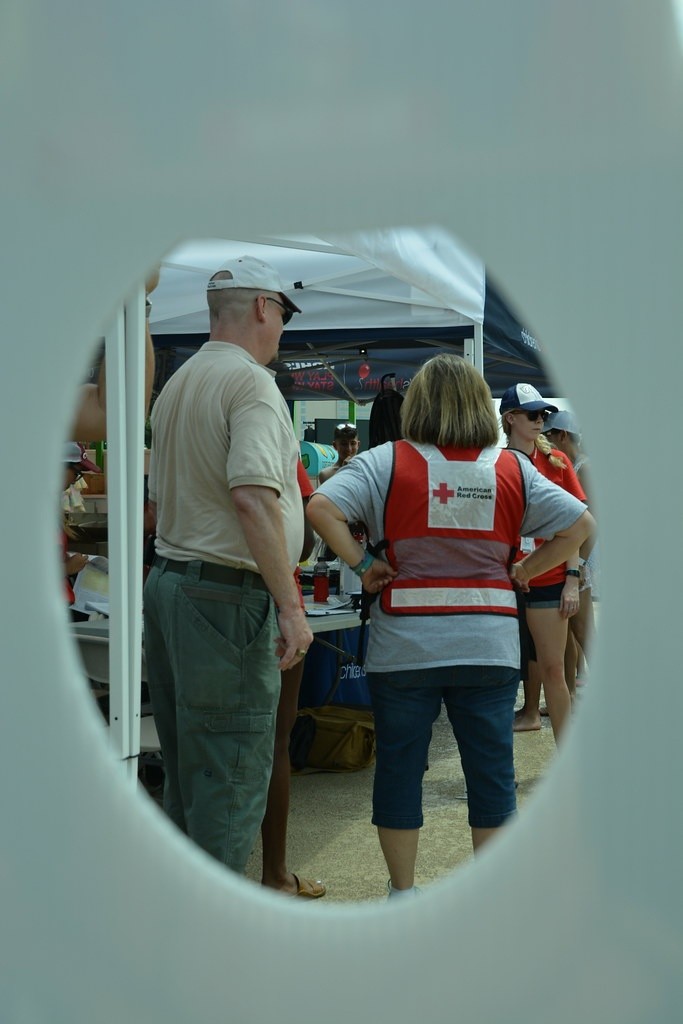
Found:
[297,649,305,658]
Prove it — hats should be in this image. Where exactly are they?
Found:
[499,382,558,415]
[64,440,102,473]
[206,255,302,313]
[539,410,582,435]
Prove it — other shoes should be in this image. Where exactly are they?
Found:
[261,871,326,899]
[539,705,550,716]
[385,877,422,903]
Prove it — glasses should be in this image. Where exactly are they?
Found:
[66,462,83,482]
[512,411,549,422]
[335,423,356,440]
[266,297,293,325]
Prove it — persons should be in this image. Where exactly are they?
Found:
[500,383,587,746]
[319,424,358,485]
[312,356,593,891]
[63,442,102,492]
[144,257,325,898]
[68,260,164,438]
[540,409,591,666]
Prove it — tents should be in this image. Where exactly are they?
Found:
[98,229,558,783]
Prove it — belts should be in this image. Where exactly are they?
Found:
[152,549,268,592]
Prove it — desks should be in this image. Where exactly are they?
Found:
[262,596,376,888]
[68,616,155,685]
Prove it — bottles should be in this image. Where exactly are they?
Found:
[314,557,329,603]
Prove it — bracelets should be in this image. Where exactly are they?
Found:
[567,570,581,577]
[349,550,374,575]
